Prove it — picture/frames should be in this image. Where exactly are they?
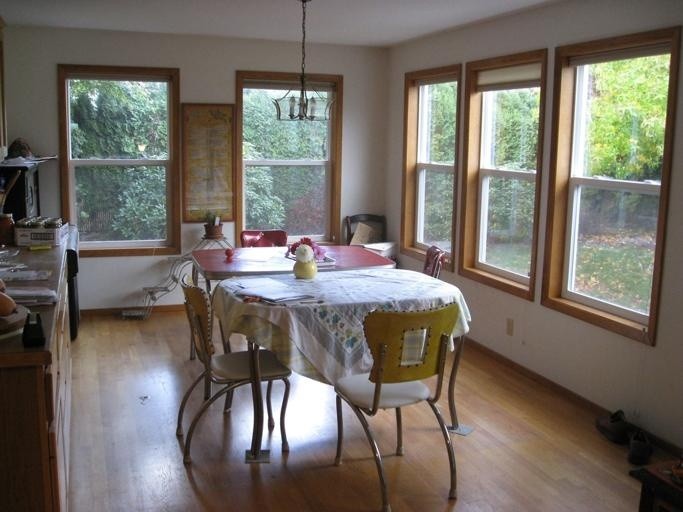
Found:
[179,101,235,225]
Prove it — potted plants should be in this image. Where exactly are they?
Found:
[202,209,225,239]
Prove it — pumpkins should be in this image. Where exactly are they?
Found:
[0,279,17,316]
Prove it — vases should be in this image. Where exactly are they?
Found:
[292,259,316,279]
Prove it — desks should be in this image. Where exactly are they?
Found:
[66,224,82,342]
[189,245,396,414]
[206,268,469,461]
[625,458,682,510]
[0,244,75,512]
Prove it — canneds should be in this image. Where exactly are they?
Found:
[0,213,17,244]
[18,216,62,228]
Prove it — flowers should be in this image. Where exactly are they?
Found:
[288,235,326,260]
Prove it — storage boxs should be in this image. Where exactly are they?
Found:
[14,222,69,249]
[362,241,397,262]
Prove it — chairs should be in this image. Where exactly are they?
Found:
[344,215,388,255]
[421,244,447,279]
[240,229,288,249]
[335,301,462,512]
[175,270,291,460]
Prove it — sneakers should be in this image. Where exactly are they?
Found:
[596,410,627,440]
[626,430,652,464]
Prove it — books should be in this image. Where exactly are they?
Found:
[5,287,57,307]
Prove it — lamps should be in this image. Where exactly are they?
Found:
[272,0,332,120]
